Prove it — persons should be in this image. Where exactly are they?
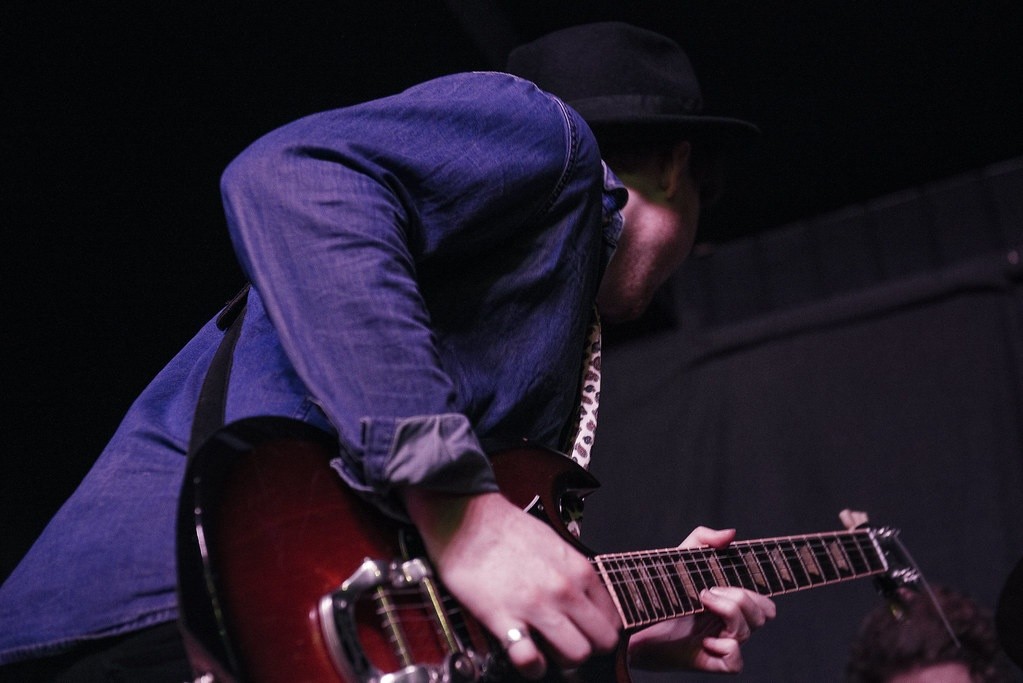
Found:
[1,21,776,683]
[841,588,998,683]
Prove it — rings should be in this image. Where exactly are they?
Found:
[501,628,531,650]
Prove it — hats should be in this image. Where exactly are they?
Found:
[506,22,763,163]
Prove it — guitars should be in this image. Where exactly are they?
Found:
[171,408,923,683]
